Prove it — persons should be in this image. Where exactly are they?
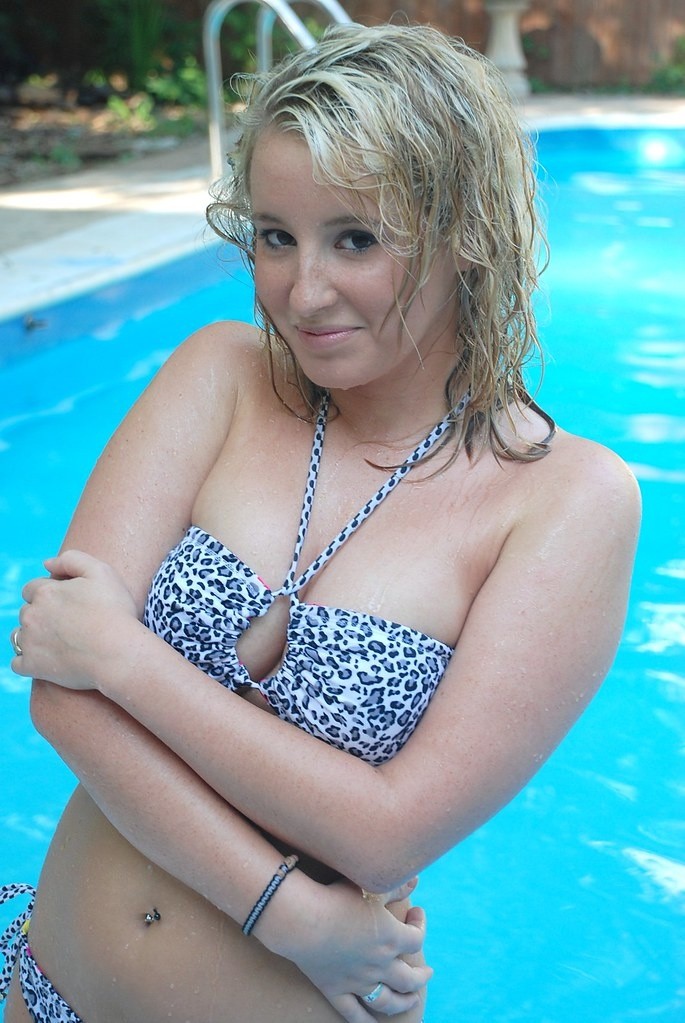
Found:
[0,21,646,1023]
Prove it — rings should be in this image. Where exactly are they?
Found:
[361,983,384,1004]
[13,630,21,656]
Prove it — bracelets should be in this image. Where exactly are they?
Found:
[241,853,301,937]
